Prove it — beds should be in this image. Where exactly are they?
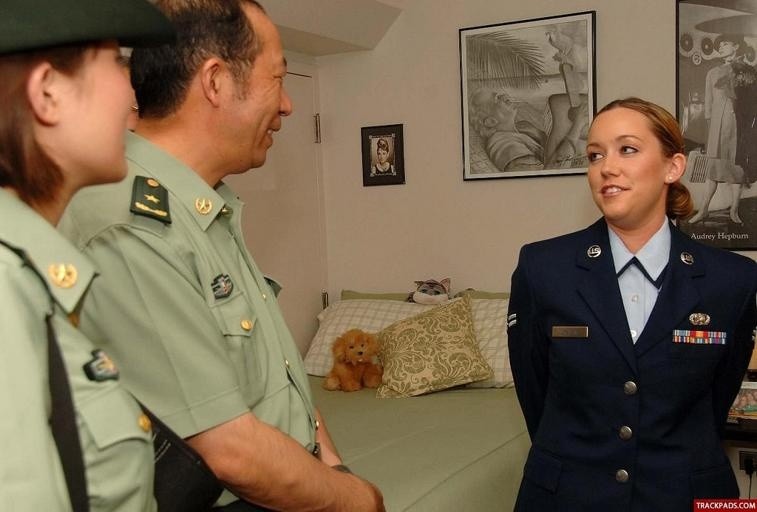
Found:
[303,290,535,512]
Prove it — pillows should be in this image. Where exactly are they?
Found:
[302,298,516,400]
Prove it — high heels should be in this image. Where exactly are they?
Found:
[688,214,709,225]
[729,213,745,228]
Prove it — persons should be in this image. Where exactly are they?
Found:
[404,277,451,305]
[686,33,757,227]
[466,79,587,172]
[506,97,757,512]
[372,138,396,176]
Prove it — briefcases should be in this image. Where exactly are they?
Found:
[683,147,710,184]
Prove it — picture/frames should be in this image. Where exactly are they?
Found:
[458,8,597,181]
[672,1,757,255]
[359,122,408,188]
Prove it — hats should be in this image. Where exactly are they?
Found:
[377,139,388,150]
[0,0,173,52]
[713,32,747,51]
